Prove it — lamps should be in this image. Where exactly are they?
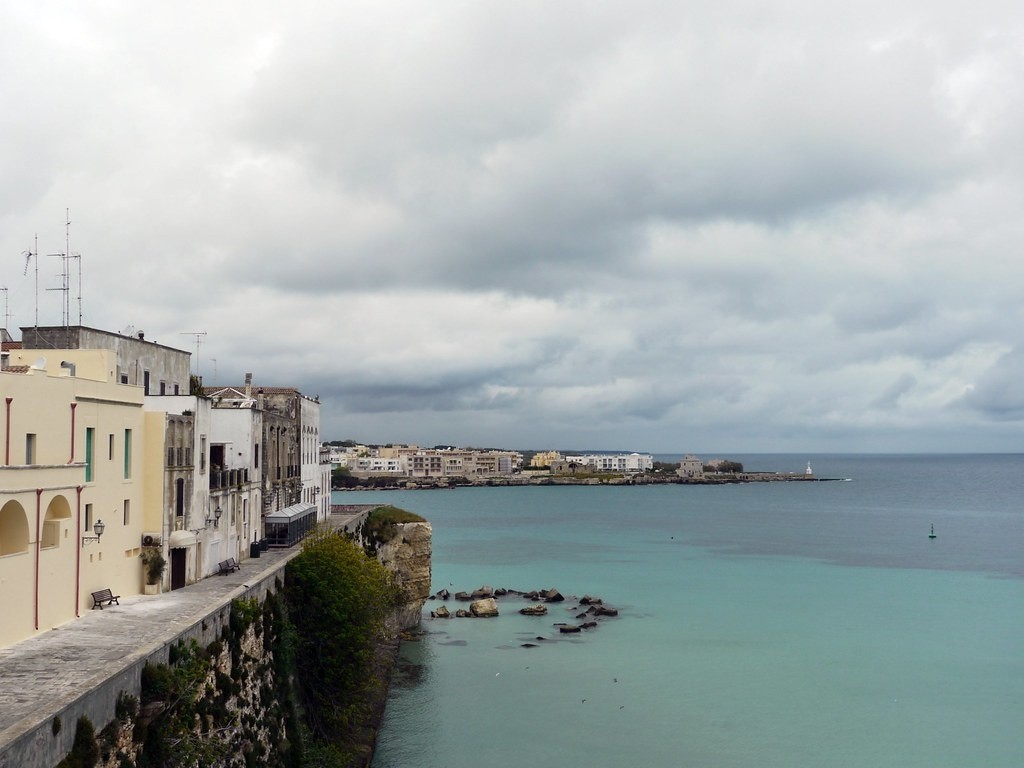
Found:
[312,486,321,495]
[83,519,105,543]
[207,506,223,526]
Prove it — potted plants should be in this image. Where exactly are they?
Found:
[144,555,167,595]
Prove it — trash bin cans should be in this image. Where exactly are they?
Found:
[261,538,268,553]
[250,541,261,558]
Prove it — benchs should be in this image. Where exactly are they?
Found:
[91,588,121,610]
[218,557,240,577]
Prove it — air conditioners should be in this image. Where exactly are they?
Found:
[142,533,163,546]
